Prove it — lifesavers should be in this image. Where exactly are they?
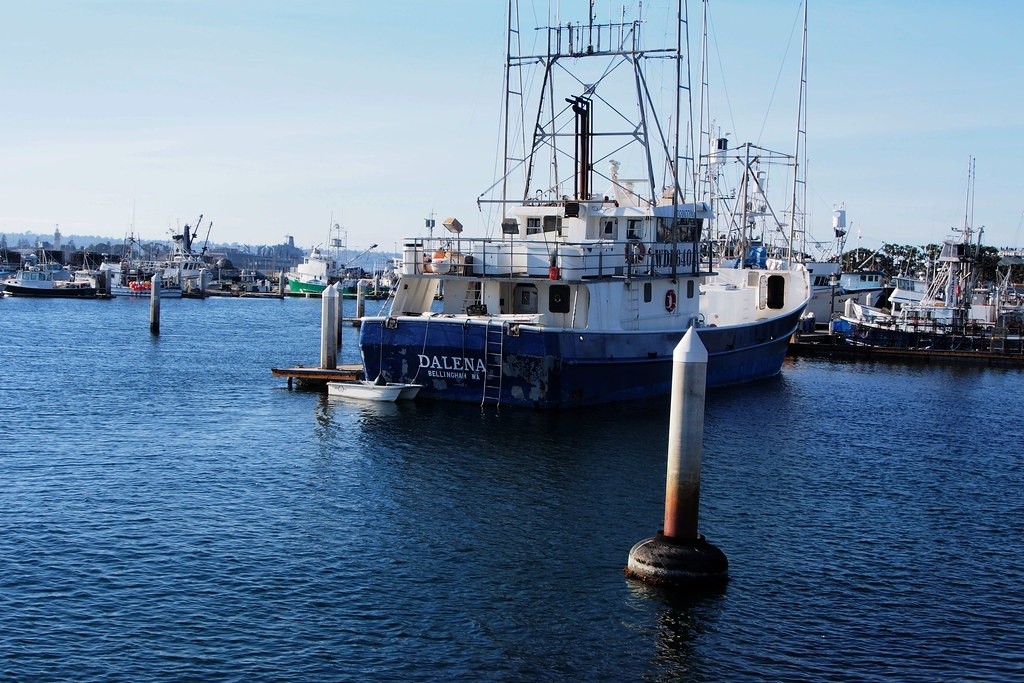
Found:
[664,289,677,313]
[624,239,646,264]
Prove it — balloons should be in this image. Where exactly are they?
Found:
[128,281,151,290]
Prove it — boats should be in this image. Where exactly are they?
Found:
[722,149,1023,363]
[346,0,811,419]
[378,242,446,301]
[228,269,271,293]
[0,242,101,299]
[94,233,185,299]
[158,214,217,299]
[284,211,388,301]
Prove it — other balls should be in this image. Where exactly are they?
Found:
[128,280,151,290]
